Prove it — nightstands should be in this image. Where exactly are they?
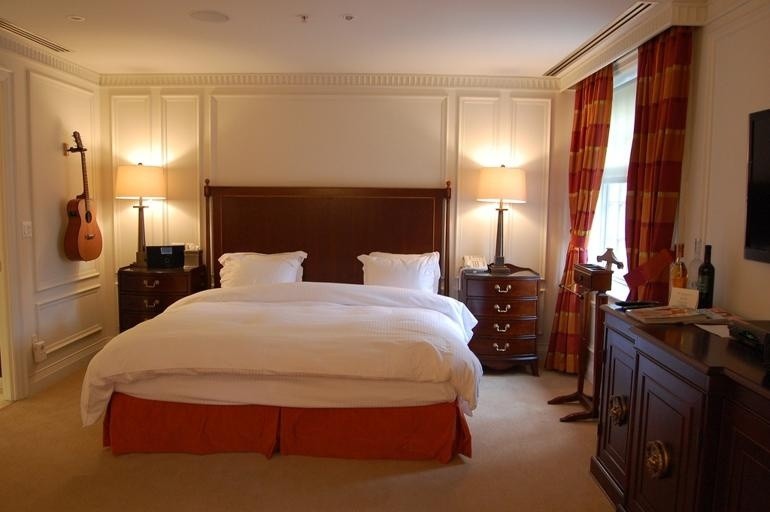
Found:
[117,266,208,335]
[455,262,543,378]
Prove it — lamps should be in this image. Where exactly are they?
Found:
[113,161,168,269]
[475,162,529,275]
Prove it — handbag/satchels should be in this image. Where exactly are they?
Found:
[146,245,184,268]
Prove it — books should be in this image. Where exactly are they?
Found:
[626,303,743,325]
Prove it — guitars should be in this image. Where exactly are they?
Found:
[64,131,102,260]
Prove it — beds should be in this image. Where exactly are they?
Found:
[79,175,484,466]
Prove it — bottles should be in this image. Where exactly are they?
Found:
[670,243,688,290]
[696,244,715,310]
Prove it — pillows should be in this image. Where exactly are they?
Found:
[354,249,442,296]
[216,250,309,287]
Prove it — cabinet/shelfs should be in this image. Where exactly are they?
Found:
[588,302,769,511]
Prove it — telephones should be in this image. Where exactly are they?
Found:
[462,255,488,271]
